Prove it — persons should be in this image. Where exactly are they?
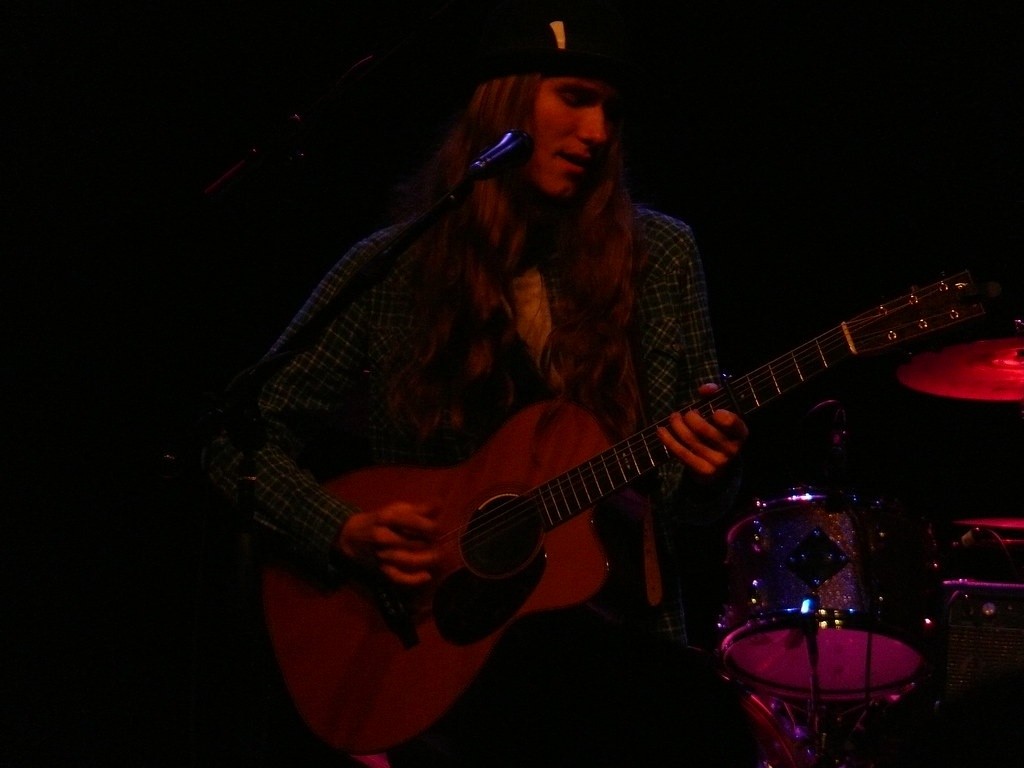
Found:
[198,1,749,768]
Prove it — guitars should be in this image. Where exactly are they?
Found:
[262,266,995,756]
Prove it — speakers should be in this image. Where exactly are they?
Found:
[945,592,1023,738]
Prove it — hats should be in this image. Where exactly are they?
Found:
[470,0,635,99]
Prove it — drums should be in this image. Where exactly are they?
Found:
[720,492,931,703]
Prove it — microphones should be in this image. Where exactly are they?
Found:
[828,407,848,455]
[959,527,983,547]
[470,130,534,180]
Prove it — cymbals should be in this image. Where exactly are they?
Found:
[896,336,1024,402]
[953,518,1024,531]
